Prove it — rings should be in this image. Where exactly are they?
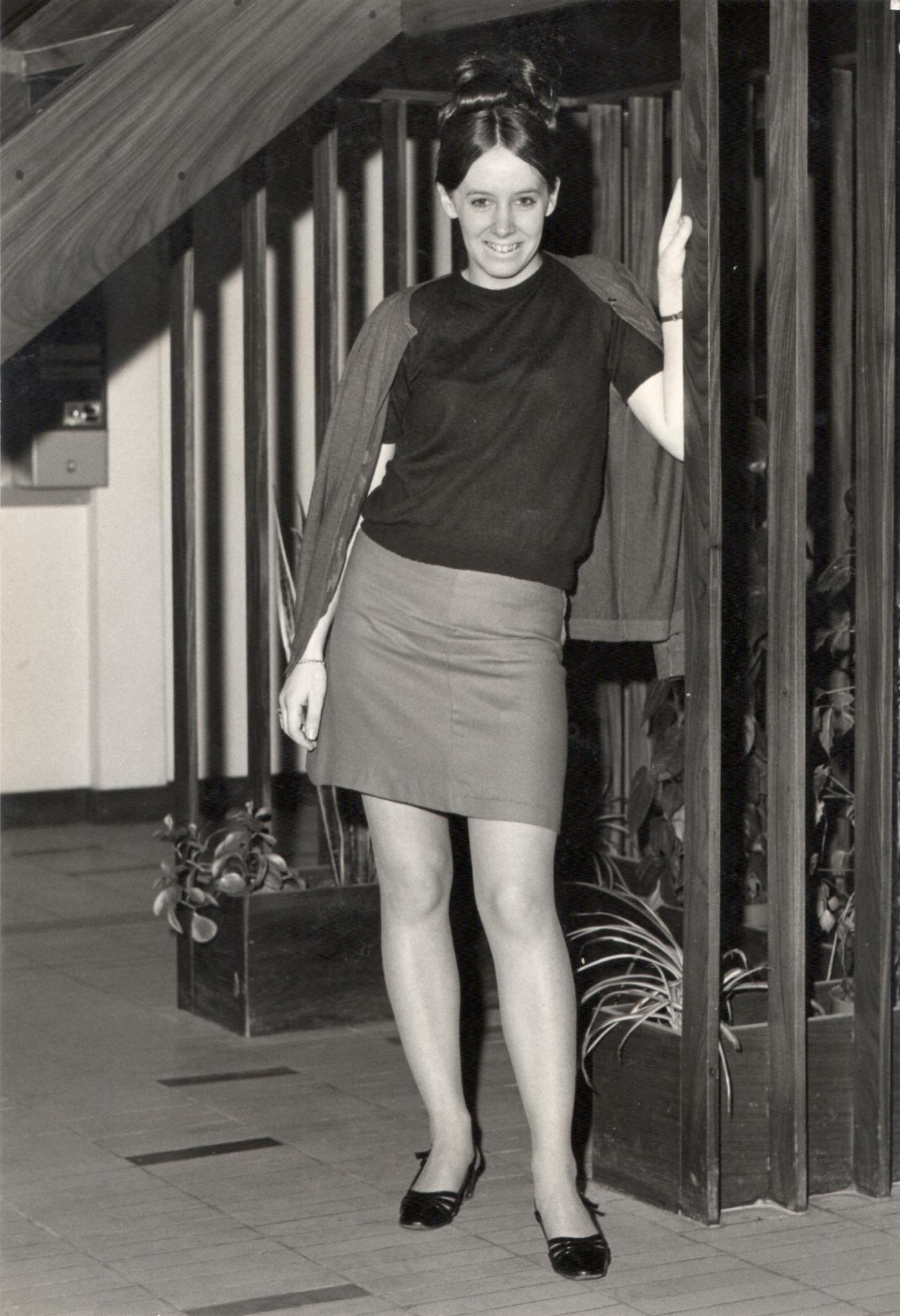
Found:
[276,708,284,719]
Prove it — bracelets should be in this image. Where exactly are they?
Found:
[659,311,684,324]
[294,657,327,670]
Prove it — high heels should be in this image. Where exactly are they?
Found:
[534,1194,610,1280]
[398,1145,487,1230]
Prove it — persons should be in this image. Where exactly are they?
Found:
[269,48,693,1286]
